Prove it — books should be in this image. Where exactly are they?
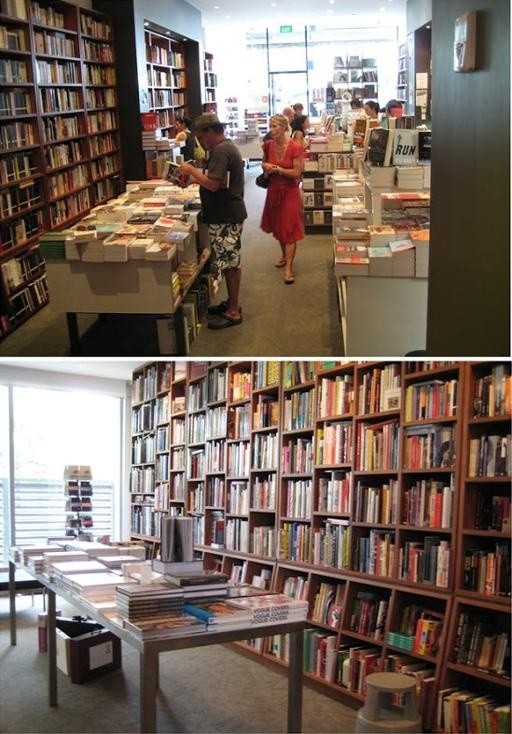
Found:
[10,360,511,733]
[302,55,431,279]
[204,168,230,189]
[0,0,222,354]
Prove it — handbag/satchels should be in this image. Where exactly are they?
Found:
[255,172,271,189]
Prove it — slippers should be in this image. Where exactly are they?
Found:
[275,256,287,267]
[283,267,296,284]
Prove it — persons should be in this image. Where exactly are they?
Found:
[292,102,304,119]
[180,112,248,331]
[260,112,306,285]
[291,115,311,144]
[262,105,297,141]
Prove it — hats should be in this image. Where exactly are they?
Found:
[188,112,229,139]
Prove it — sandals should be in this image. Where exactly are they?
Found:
[207,300,242,314]
[207,312,243,329]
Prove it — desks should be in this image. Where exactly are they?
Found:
[8,540,307,733]
